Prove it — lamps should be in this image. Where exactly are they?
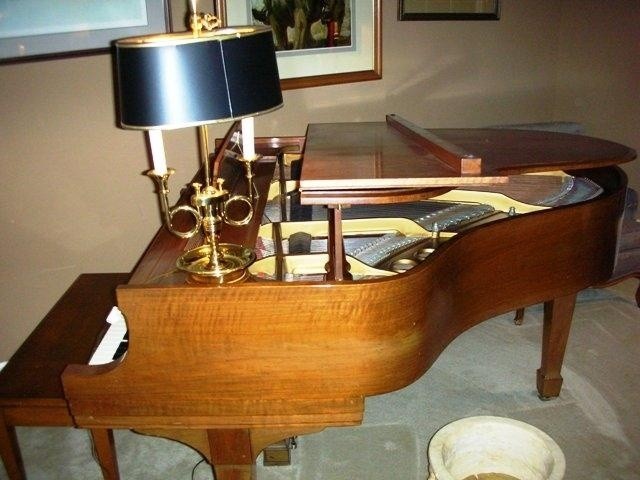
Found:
[111,13,285,285]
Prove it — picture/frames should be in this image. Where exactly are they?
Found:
[214,0,383,92]
[0,0,173,66]
[398,0,501,20]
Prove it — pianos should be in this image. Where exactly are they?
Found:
[62,115,637,480]
